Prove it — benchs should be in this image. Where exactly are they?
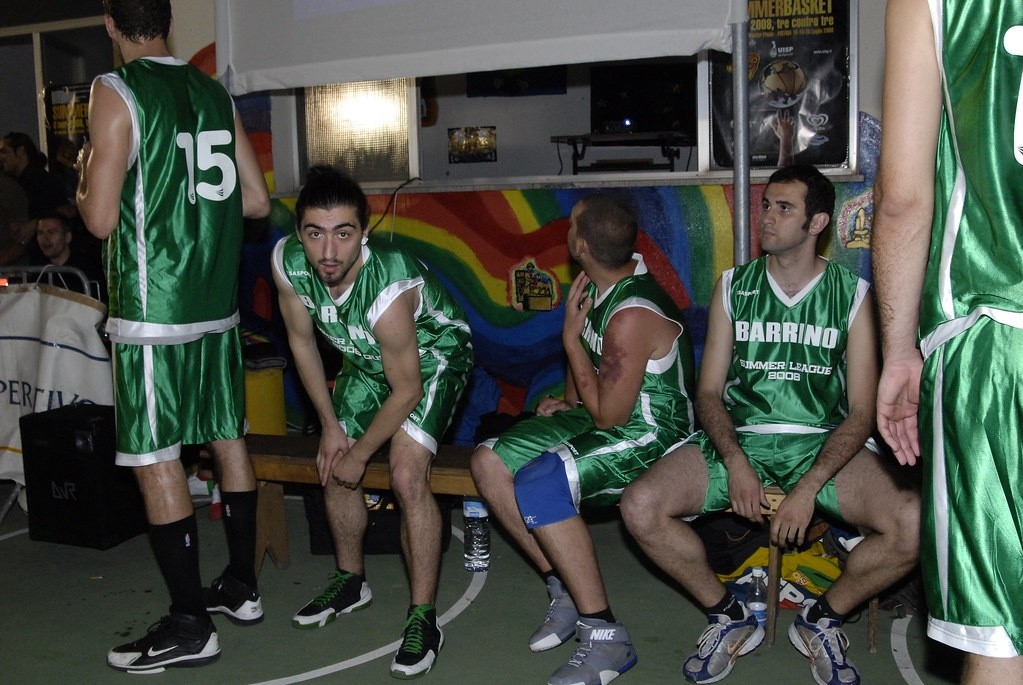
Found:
[200,434,789,648]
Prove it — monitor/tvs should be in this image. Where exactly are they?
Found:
[588,54,699,137]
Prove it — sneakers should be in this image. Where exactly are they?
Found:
[546,617,638,685]
[208,481,223,520]
[169,573,265,627]
[105,612,222,675]
[291,569,373,630]
[786,600,862,685]
[527,575,580,653]
[389,603,443,679]
[186,472,214,502]
[681,601,764,685]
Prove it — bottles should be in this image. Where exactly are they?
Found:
[462,496,492,572]
[746,567,768,629]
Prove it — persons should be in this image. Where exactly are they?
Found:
[620,165,923,684]
[271,167,474,680]
[1,131,108,316]
[468,194,696,685]
[76,0,270,675]
[871,1,1023,684]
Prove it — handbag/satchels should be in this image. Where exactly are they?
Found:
[690,510,929,621]
[305,479,451,557]
